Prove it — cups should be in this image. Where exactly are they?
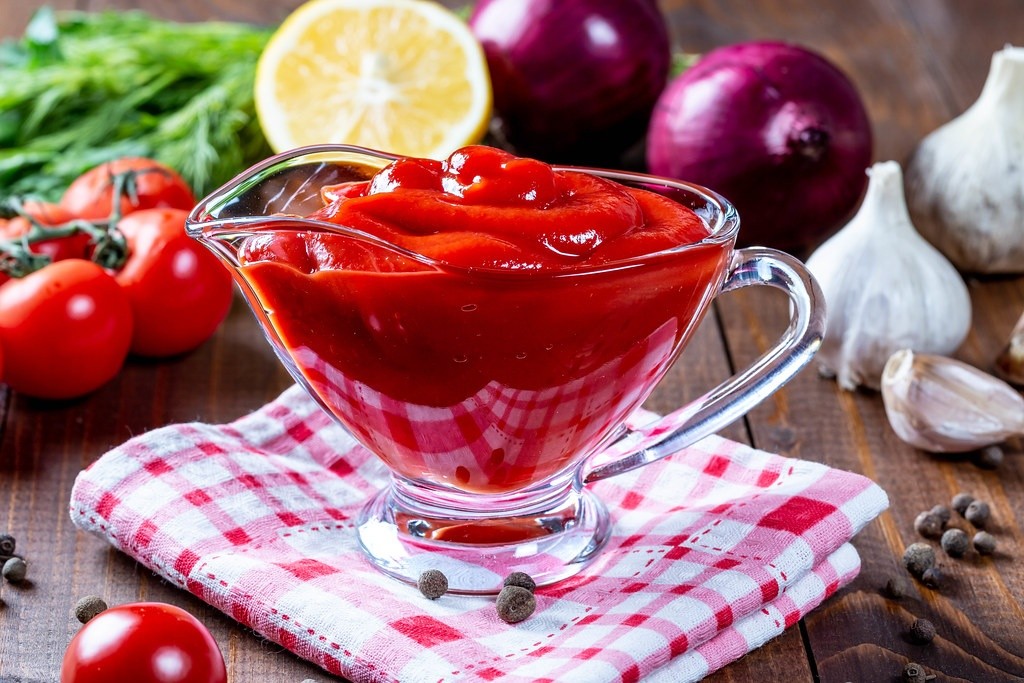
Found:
[186,141,825,596]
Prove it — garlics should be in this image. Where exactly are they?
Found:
[803,42,1024,455]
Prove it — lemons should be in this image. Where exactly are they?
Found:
[254,0,492,180]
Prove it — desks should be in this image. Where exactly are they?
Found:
[0,0,1023,683]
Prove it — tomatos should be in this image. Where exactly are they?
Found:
[0,156,234,682]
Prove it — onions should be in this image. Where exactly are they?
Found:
[466,0,876,262]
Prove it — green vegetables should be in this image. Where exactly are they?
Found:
[0,2,272,216]
